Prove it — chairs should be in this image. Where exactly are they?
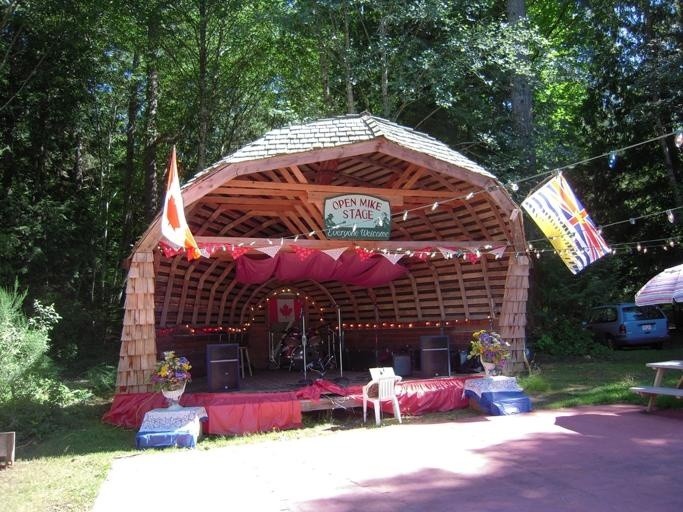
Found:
[361,376,401,426]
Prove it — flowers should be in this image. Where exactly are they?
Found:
[145,347,192,395]
[465,327,512,370]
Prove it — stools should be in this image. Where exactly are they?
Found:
[236,346,253,379]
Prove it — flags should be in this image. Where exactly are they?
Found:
[161,146,200,260]
[268,298,303,323]
[524,175,613,274]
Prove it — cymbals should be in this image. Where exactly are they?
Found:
[318,319,338,332]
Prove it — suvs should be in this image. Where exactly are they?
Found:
[582,301,670,351]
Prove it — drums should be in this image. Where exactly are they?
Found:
[285,327,306,346]
[305,326,322,345]
[283,342,306,369]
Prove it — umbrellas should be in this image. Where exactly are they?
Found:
[634,264,683,306]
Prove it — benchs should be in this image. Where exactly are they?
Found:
[627,383,682,416]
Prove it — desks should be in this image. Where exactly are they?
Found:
[640,359,682,415]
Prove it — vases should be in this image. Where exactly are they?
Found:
[478,353,505,377]
[157,378,188,409]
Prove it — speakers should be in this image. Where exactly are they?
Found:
[419,335,451,377]
[206,344,240,391]
